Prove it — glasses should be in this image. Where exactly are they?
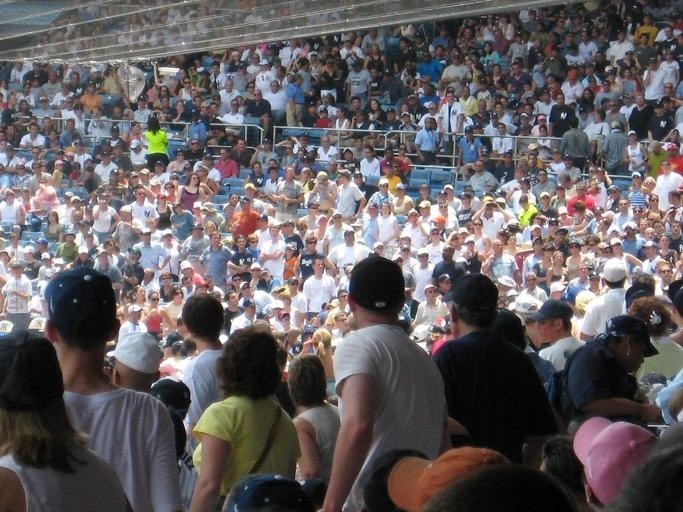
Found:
[250,86,255,88]
[308,241,317,245]
[402,249,410,252]
[184,81,191,84]
[162,278,169,280]
[254,94,261,96]
[647,218,654,220]
[213,98,220,100]
[551,223,558,226]
[429,290,436,293]
[474,224,482,226]
[175,292,183,296]
[569,245,579,249]
[193,177,199,180]
[440,206,448,209]
[575,188,585,191]
[250,241,259,244]
[539,174,546,176]
[399,148,406,150]
[151,298,159,302]
[242,286,250,290]
[432,233,439,235]
[191,143,200,145]
[190,68,196,71]
[161,90,167,92]
[634,210,641,213]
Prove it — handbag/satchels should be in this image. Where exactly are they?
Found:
[546,369,575,426]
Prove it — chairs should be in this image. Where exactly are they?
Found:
[212,166,268,216]
[282,127,327,145]
[1,221,63,255]
[315,160,331,174]
[406,167,482,202]
[16,150,32,160]
[241,118,262,145]
[269,109,286,132]
[613,179,632,190]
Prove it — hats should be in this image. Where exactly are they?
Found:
[431,216,446,223]
[632,172,642,178]
[63,192,74,197]
[137,96,147,101]
[593,206,603,212]
[193,201,203,209]
[35,239,48,248]
[140,169,150,174]
[605,315,659,357]
[368,203,379,208]
[193,278,210,288]
[41,253,50,260]
[319,108,327,113]
[654,102,663,108]
[482,196,494,203]
[111,126,119,131]
[175,149,183,156]
[271,300,284,308]
[373,242,383,248]
[589,269,600,279]
[64,151,74,157]
[549,217,559,224]
[221,475,326,512]
[129,171,138,177]
[161,229,173,237]
[517,177,531,183]
[251,262,261,269]
[203,202,218,212]
[260,268,270,276]
[531,225,542,232]
[498,276,515,287]
[417,249,430,255]
[397,184,404,190]
[643,241,658,247]
[106,333,162,374]
[444,184,454,190]
[22,246,34,254]
[78,218,94,227]
[0,330,63,411]
[568,240,579,247]
[388,447,511,512]
[603,258,626,282]
[496,197,507,203]
[586,261,594,269]
[198,164,209,175]
[272,287,285,293]
[611,120,621,129]
[257,214,267,224]
[610,238,622,245]
[350,258,405,312]
[191,222,203,231]
[528,143,539,151]
[401,112,411,117]
[55,160,63,166]
[240,195,250,203]
[130,140,140,149]
[314,172,327,184]
[128,305,142,314]
[622,221,638,231]
[239,282,249,290]
[400,233,412,240]
[332,209,342,217]
[337,169,352,180]
[670,280,683,319]
[533,237,543,243]
[379,180,389,185]
[430,226,439,232]
[418,200,431,208]
[438,191,447,196]
[424,285,437,297]
[607,185,619,192]
[458,227,468,234]
[180,260,193,272]
[442,274,498,312]
[284,220,294,226]
[96,248,106,256]
[465,126,473,133]
[238,62,245,69]
[438,274,454,284]
[71,196,81,203]
[63,230,76,239]
[550,229,568,242]
[401,244,410,250]
[539,192,550,199]
[141,228,151,235]
[128,247,142,259]
[151,375,191,412]
[45,267,115,331]
[244,299,259,307]
[628,131,637,137]
[528,300,573,320]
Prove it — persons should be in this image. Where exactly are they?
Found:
[493,309,560,393]
[315,298,339,326]
[343,312,357,337]
[537,433,588,512]
[668,278,683,349]
[287,355,341,483]
[654,253,683,299]
[562,315,664,441]
[275,328,301,356]
[150,378,198,511]
[632,272,658,300]
[411,321,455,354]
[508,273,548,318]
[433,275,570,475]
[0,328,133,511]
[181,294,230,459]
[566,263,591,306]
[189,323,302,512]
[411,284,451,328]
[316,256,452,512]
[159,317,197,377]
[224,263,308,334]
[106,333,164,393]
[179,261,226,305]
[523,318,551,353]
[628,298,683,403]
[323,289,352,334]
[330,312,349,342]
[579,256,631,342]
[105,248,183,331]
[497,275,518,310]
[529,299,587,372]
[42,265,184,512]
[1,1,683,281]
[575,271,604,316]
[301,328,337,379]
